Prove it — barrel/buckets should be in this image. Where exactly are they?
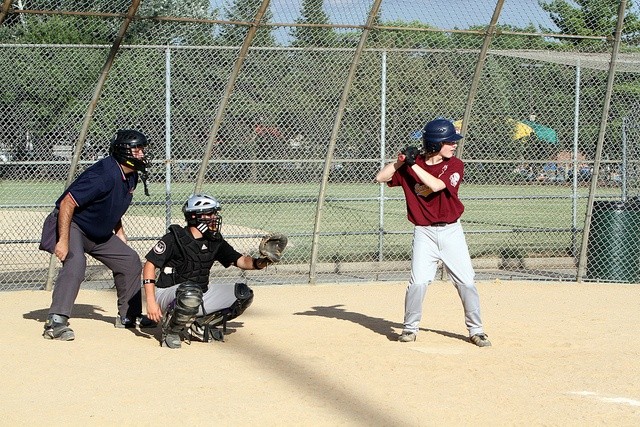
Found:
[585,199,640,285]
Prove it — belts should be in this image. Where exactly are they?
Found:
[431,219,457,226]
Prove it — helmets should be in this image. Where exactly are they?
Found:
[181,193,223,241]
[109,129,151,173]
[423,119,464,155]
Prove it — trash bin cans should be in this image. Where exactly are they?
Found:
[588,199,636,280]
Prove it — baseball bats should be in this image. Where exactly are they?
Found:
[398,147,425,161]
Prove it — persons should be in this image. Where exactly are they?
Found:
[40,130,157,341]
[143,194,280,348]
[376,119,491,348]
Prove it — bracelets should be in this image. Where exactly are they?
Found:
[143,279,157,285]
[253,258,262,270]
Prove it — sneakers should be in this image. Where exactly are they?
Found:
[161,328,181,348]
[398,331,416,342]
[470,333,492,348]
[188,321,216,343]
[115,311,157,329]
[44,314,75,341]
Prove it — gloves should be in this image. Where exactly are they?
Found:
[401,147,421,162]
[401,149,416,167]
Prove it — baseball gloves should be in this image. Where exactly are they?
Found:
[258,235,288,264]
